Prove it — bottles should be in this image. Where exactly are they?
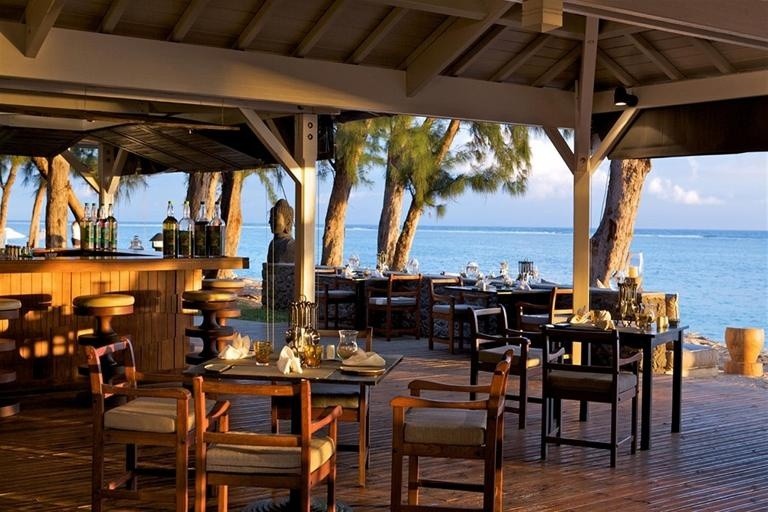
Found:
[196,199,209,257]
[163,202,179,258]
[90,201,96,247]
[207,202,225,256]
[82,201,95,255]
[108,201,119,251]
[97,203,106,252]
[179,203,194,259]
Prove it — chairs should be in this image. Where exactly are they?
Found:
[314,267,360,329]
[428,276,491,354]
[83,335,232,512]
[467,303,564,430]
[538,324,643,469]
[516,286,573,363]
[191,375,344,512]
[270,327,374,489]
[388,349,516,511]
[367,273,424,342]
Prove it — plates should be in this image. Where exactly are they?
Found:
[203,363,231,373]
[339,366,385,376]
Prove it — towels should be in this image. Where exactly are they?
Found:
[277,345,304,375]
[342,349,386,368]
[596,309,617,330]
[568,304,590,324]
[217,344,250,360]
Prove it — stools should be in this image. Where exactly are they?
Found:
[202,279,245,354]
[0,298,22,419]
[72,293,135,408]
[180,289,238,395]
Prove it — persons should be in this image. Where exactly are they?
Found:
[267,200,296,262]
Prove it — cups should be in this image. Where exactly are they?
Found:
[254,342,273,366]
[304,345,323,367]
[639,303,657,330]
[627,252,644,312]
